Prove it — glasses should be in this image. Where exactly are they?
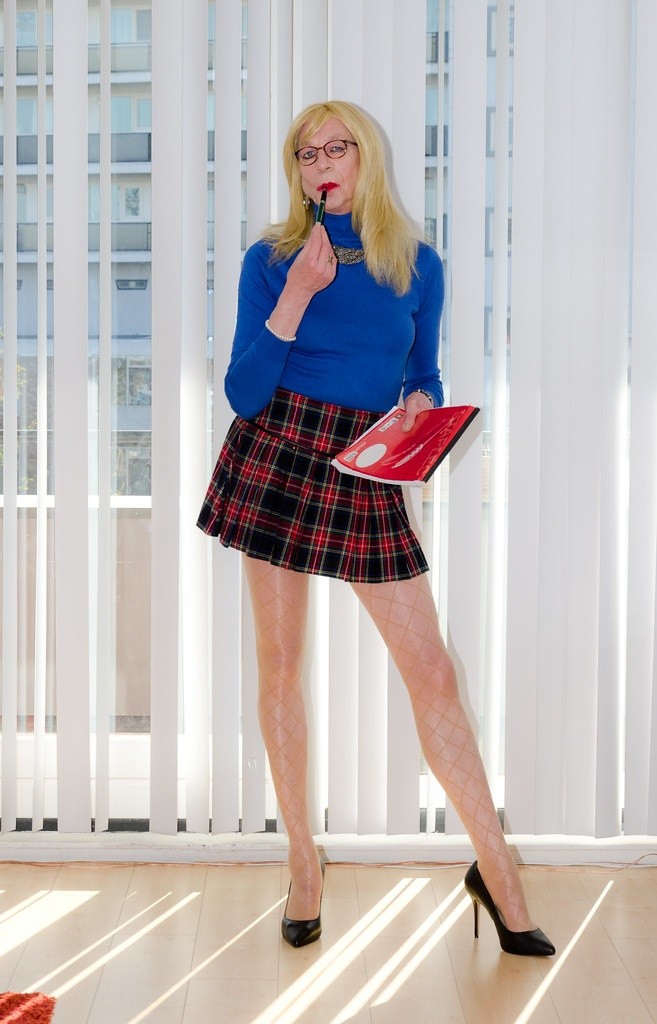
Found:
[294,139,359,166]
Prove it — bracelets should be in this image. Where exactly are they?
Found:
[264,319,298,344]
[413,388,436,404]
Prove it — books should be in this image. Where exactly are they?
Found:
[331,404,480,486]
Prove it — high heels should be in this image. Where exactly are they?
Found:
[465,859,556,957]
[282,855,326,948]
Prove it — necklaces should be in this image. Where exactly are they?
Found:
[331,244,365,265]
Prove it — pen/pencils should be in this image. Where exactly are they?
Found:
[315,190,327,222]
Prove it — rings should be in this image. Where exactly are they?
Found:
[325,255,335,266]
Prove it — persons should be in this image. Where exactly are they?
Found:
[195,100,558,958]
